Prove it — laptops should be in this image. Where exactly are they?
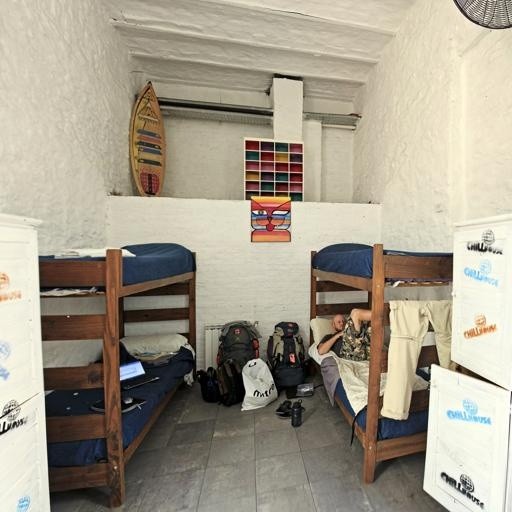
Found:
[118,359,160,390]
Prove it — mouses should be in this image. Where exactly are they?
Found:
[123,397,132,403]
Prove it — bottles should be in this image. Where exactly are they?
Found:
[291,398,303,427]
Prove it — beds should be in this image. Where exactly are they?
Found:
[39,244,198,509]
[307,243,452,485]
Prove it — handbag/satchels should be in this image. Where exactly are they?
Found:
[196,358,279,411]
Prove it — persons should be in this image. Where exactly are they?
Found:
[317,308,372,360]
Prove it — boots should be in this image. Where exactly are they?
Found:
[276,400,306,427]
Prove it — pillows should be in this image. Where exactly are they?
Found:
[118,333,187,354]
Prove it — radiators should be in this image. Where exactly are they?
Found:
[205,325,224,372]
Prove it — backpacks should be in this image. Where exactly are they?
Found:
[215,321,307,406]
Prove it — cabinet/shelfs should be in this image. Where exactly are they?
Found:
[1,214,52,512]
[420,216,512,511]
[244,136,304,202]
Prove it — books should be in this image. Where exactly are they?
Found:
[60,247,136,260]
[93,395,146,415]
